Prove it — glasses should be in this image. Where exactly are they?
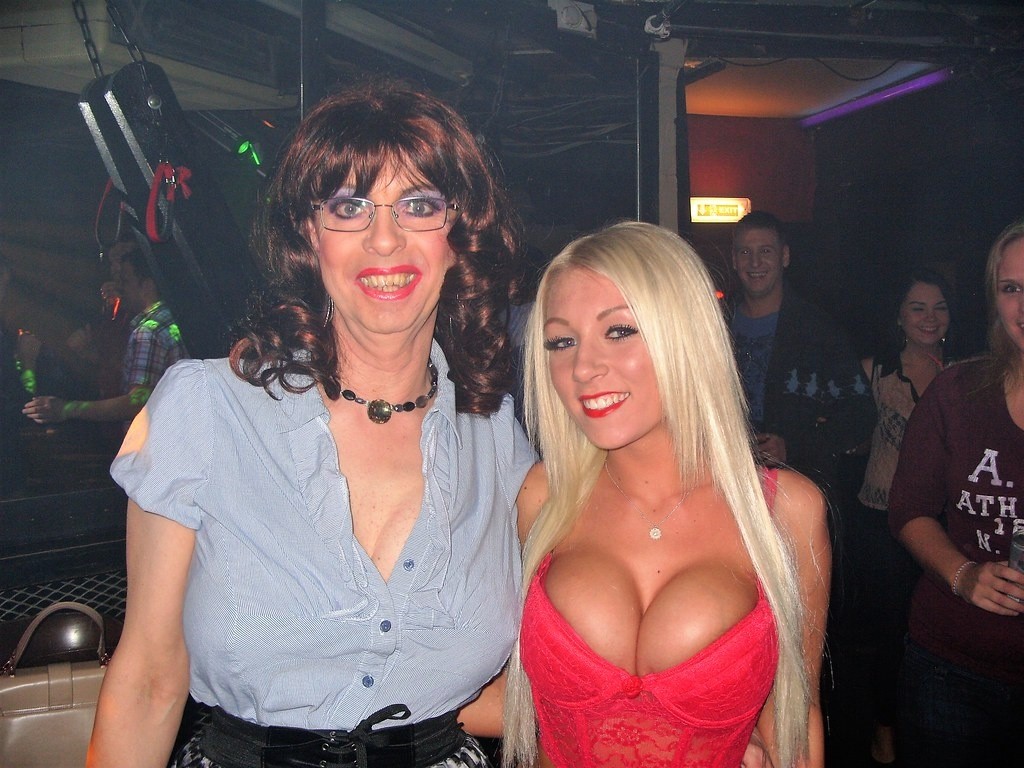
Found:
[311,195,460,233]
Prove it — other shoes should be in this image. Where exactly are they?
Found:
[871,718,896,763]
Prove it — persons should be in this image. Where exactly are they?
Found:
[87,77,542,768]
[0,210,1024,768]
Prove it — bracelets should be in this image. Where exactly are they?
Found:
[952,561,977,596]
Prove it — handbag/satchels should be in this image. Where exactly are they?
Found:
[0,602,113,768]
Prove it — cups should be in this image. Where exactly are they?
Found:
[1008,530,1024,603]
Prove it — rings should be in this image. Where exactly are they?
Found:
[766,432,770,440]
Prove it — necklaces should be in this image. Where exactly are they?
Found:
[331,358,439,425]
[605,460,697,540]
